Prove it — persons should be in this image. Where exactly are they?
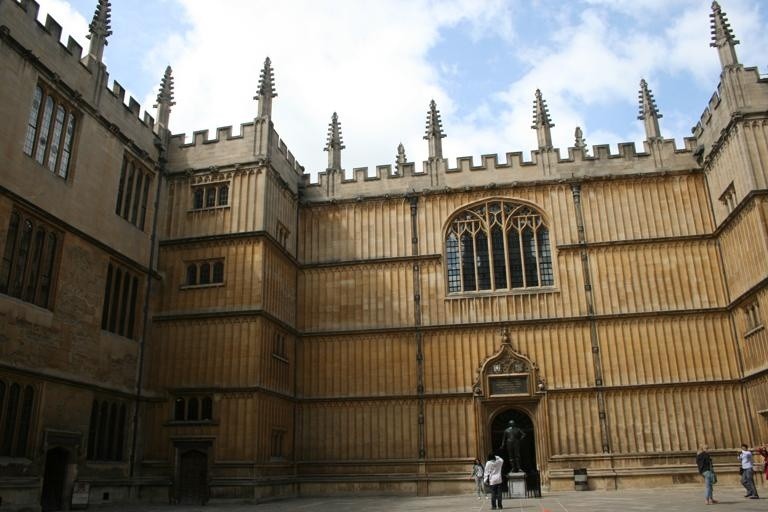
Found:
[497,419,526,473]
[758,443,768,479]
[736,444,758,499]
[483,452,504,509]
[695,442,719,504]
[468,457,488,499]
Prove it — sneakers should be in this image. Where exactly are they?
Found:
[705,501,712,505]
[709,500,718,503]
[744,493,751,497]
[750,495,759,499]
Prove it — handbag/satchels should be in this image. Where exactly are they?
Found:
[740,467,744,475]
[710,461,717,484]
